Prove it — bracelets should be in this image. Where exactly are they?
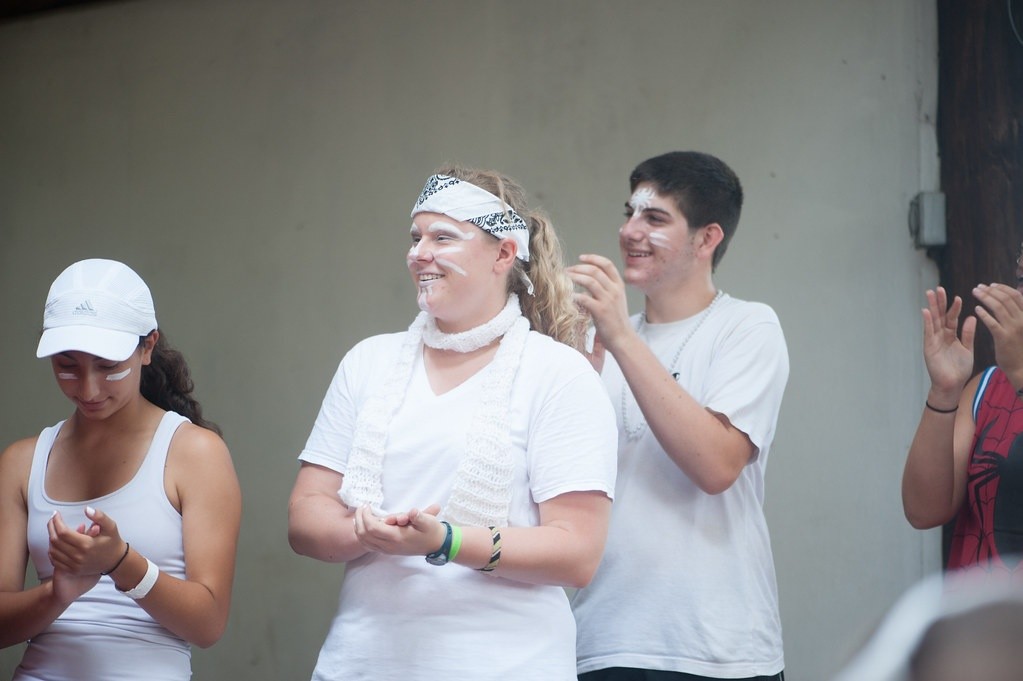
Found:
[100,543,130,575]
[474,526,502,572]
[114,556,160,600]
[448,525,462,562]
[926,400,959,413]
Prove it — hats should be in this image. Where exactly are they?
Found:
[36,258,158,362]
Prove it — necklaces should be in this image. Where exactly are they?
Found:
[622,290,723,434]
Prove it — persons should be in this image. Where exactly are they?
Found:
[551,151,786,681]
[902,250,1023,608]
[1,258,241,681]
[292,168,619,681]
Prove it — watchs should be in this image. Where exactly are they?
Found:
[424,522,452,566]
